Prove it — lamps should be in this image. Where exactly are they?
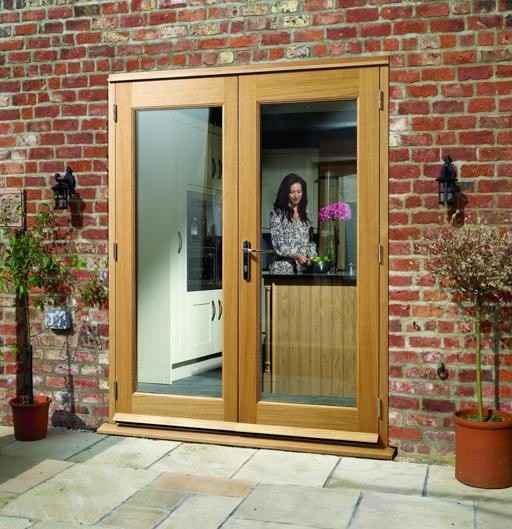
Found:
[50,167,75,210]
[436,155,461,203]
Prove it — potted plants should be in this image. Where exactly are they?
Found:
[422,215,512,490]
[0,200,108,439]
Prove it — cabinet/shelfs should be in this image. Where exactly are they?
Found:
[137,110,223,384]
[260,149,318,234]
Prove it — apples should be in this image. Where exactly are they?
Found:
[312,255,329,262]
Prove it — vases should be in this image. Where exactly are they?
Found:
[326,221,338,273]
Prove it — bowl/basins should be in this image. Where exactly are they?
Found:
[302,260,333,274]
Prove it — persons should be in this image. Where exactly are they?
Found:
[267,173,319,274]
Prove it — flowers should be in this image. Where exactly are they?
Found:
[320,201,352,272]
